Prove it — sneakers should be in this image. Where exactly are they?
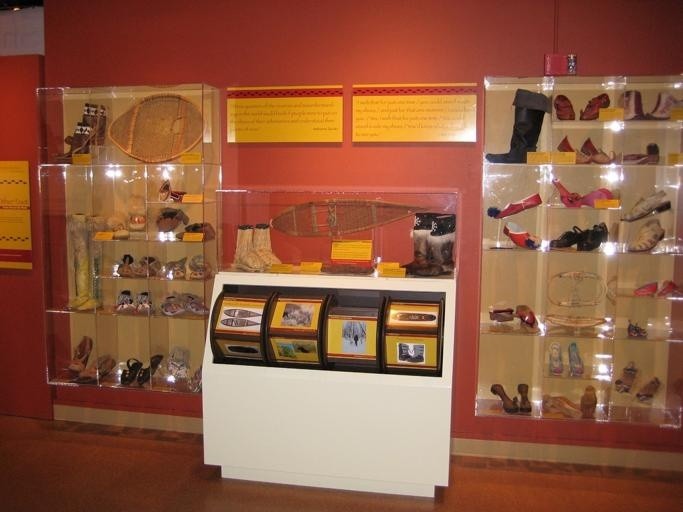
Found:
[116,253,211,280]
[111,289,209,317]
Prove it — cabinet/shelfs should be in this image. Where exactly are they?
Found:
[32,82,221,395]
[470,73,683,430]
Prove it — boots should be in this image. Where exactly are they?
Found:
[231,224,282,273]
[52,121,96,164]
[485,89,552,164]
[67,213,104,311]
[65,103,108,146]
[401,212,456,277]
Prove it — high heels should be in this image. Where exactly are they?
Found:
[488,281,677,421]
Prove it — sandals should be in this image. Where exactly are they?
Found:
[166,346,190,379]
[74,354,116,383]
[188,369,202,392]
[137,355,163,384]
[121,358,143,385]
[69,336,93,373]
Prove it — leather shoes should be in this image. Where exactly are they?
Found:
[111,179,215,241]
[553,90,673,165]
[486,179,670,252]
